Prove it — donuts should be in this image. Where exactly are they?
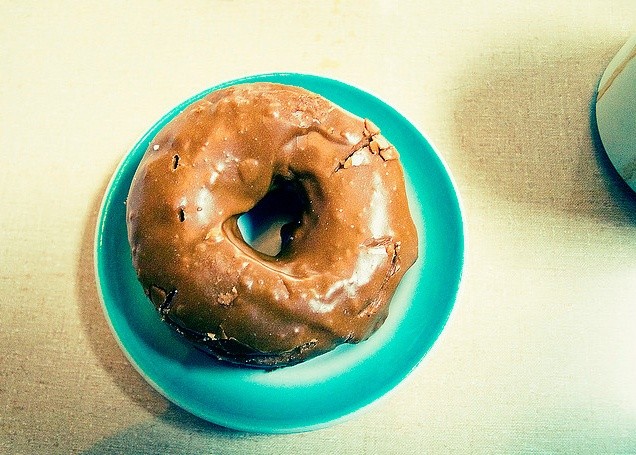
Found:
[126,82,418,372]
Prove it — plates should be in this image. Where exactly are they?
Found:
[596,31,634,193]
[92,73,465,434]
